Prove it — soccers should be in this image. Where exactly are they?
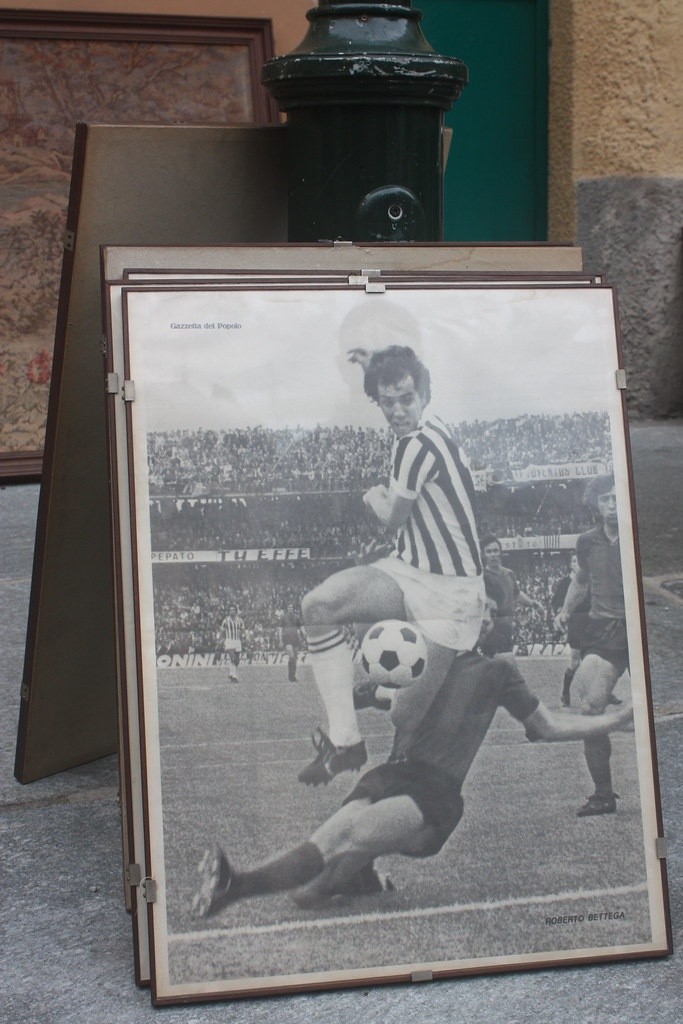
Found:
[361,617,430,689]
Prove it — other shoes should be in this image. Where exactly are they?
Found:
[576,790,619,816]
[608,694,622,705]
[288,674,298,683]
[229,673,238,683]
[561,692,570,707]
[284,870,352,910]
[525,727,544,742]
[190,843,234,926]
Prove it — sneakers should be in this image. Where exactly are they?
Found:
[298,727,367,787]
[352,680,401,711]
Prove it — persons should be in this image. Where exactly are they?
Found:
[145,341,637,918]
